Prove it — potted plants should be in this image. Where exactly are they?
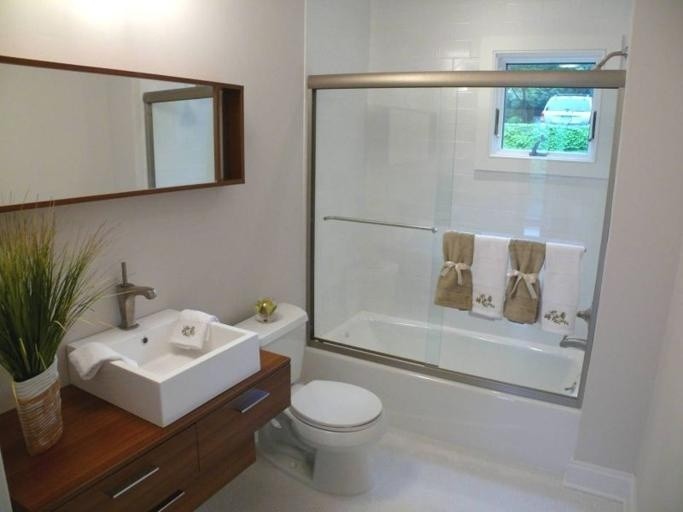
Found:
[0,188,138,457]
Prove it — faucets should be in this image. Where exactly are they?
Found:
[559,333,586,352]
[114,262,156,331]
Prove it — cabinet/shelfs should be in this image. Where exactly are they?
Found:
[0,349,290,511]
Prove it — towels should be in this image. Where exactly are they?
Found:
[434,230,586,341]
[169,308,219,352]
[68,341,136,382]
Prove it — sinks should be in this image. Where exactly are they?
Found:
[67,307,262,429]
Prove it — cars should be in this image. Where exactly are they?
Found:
[539,93,594,140]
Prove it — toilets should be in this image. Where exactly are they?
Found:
[230,302,385,497]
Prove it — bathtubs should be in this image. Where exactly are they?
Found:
[318,312,583,401]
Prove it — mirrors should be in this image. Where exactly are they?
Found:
[1,55,245,213]
